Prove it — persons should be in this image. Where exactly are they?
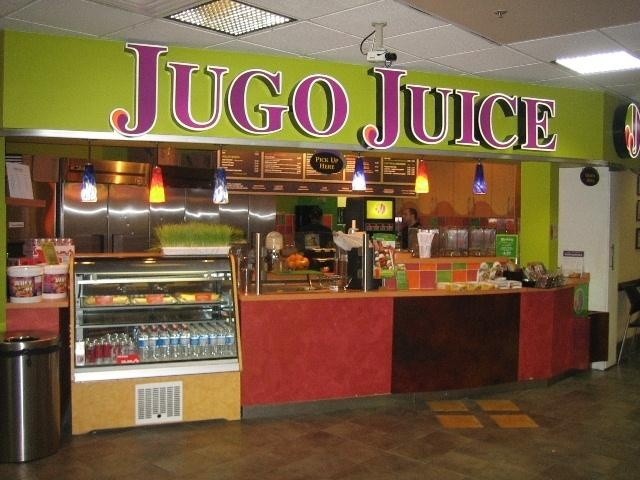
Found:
[296,206,333,249]
[399,208,423,249]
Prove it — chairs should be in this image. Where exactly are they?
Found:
[618,279,640,363]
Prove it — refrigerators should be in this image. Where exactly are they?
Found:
[343,196,401,290]
[55,157,277,268]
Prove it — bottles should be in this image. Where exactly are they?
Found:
[133,321,235,363]
[32,237,71,257]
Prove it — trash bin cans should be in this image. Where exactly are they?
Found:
[0,335,61,464]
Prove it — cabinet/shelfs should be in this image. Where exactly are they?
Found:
[68,249,243,435]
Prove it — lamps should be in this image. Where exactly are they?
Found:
[352,152,366,190]
[415,156,429,193]
[81,139,96,202]
[149,141,165,202]
[212,146,228,204]
[473,158,488,196]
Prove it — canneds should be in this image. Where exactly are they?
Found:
[82,332,137,365]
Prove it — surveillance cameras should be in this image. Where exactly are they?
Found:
[366,48,397,67]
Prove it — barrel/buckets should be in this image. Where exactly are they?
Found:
[6,264,44,305]
[41,263,71,299]
